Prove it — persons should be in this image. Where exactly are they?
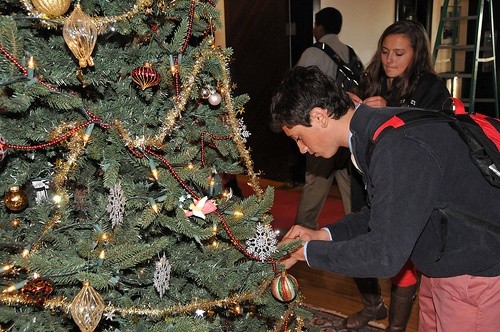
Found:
[293,6,364,231]
[270,64,500,332]
[342,18,453,331]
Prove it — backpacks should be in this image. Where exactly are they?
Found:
[311,42,362,92]
[366,98,500,188]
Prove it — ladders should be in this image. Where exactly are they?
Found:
[429,1,500,118]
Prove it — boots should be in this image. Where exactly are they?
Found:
[386,283,417,332]
[342,277,387,330]
